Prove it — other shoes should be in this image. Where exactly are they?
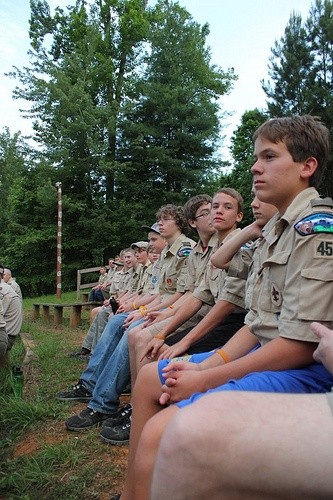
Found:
[67,347,92,362]
[119,383,132,395]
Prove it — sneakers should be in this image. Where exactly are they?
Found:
[54,379,94,399]
[65,407,113,432]
[101,402,132,429]
[98,416,131,445]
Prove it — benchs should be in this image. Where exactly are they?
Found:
[30,300,102,329]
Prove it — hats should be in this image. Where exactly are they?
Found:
[139,221,161,234]
[130,241,148,249]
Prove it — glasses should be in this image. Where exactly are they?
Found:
[194,211,209,219]
[156,217,176,223]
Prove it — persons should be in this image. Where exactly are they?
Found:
[0,265,22,360]
[55,115,333,500]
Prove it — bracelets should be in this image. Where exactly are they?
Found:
[153,335,166,340]
[132,301,136,310]
[140,311,145,317]
[169,305,174,309]
[139,305,147,310]
[215,349,229,364]
